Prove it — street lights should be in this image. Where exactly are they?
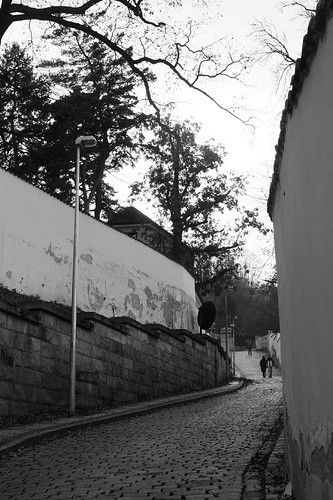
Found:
[67,135,97,417]
[222,285,238,386]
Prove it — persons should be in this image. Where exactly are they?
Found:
[266,355,274,378]
[260,356,267,378]
[247,344,252,355]
